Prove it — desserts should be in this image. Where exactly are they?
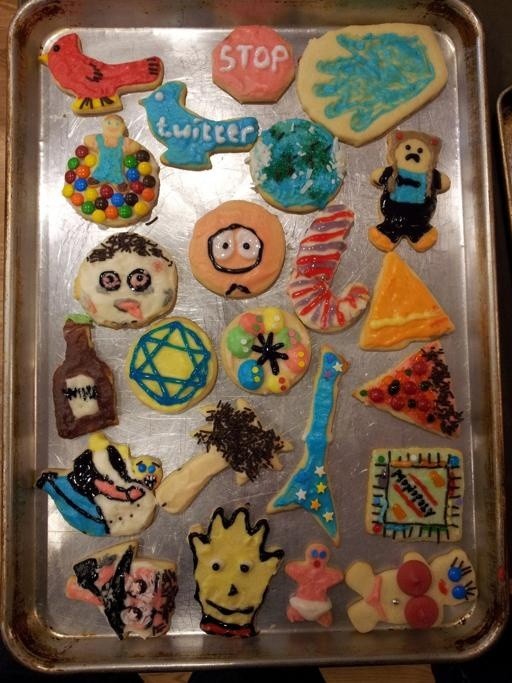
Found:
[37,25,481,642]
